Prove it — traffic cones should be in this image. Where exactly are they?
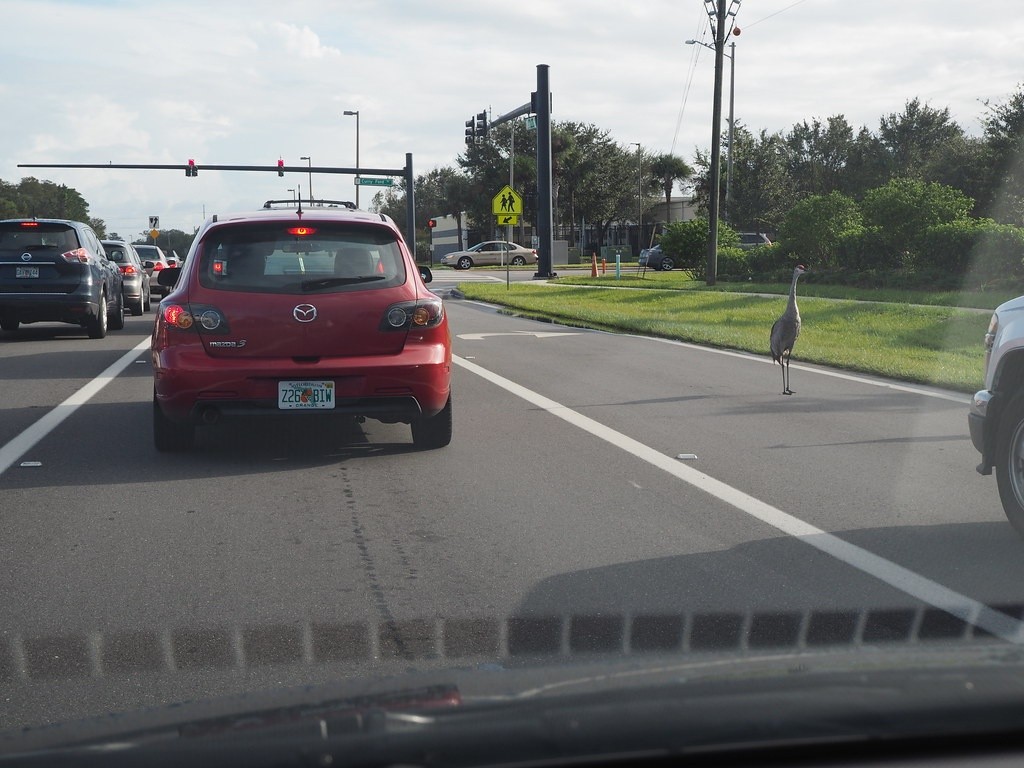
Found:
[590,252,598,277]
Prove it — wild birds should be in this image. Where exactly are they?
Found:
[769,264,810,396]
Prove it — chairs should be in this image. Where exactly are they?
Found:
[332,249,375,276]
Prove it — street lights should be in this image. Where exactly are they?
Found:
[684,39,737,228]
[343,110,359,208]
[299,157,312,206]
[287,189,295,207]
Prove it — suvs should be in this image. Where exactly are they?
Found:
[734,231,771,251]
[211,200,383,275]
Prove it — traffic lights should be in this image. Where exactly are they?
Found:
[188,159,194,167]
[428,220,436,227]
[476,110,487,137]
[192,166,198,176]
[465,115,476,149]
[185,166,191,176]
[278,159,284,176]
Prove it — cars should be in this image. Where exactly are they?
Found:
[0,218,124,339]
[150,206,453,451]
[100,240,155,316]
[638,243,694,271]
[114,244,172,299]
[440,240,537,270]
[968,293,1024,538]
[161,250,184,269]
